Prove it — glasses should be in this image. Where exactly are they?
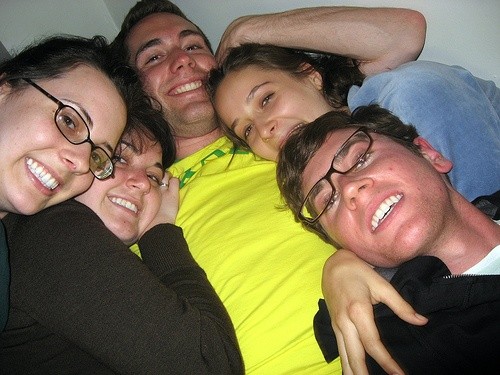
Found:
[297,125,412,240]
[18,75,114,180]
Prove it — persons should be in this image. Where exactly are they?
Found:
[0,35,245,375]
[109,0,428,375]
[205,43,500,375]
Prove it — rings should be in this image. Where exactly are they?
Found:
[160,183,168,187]
